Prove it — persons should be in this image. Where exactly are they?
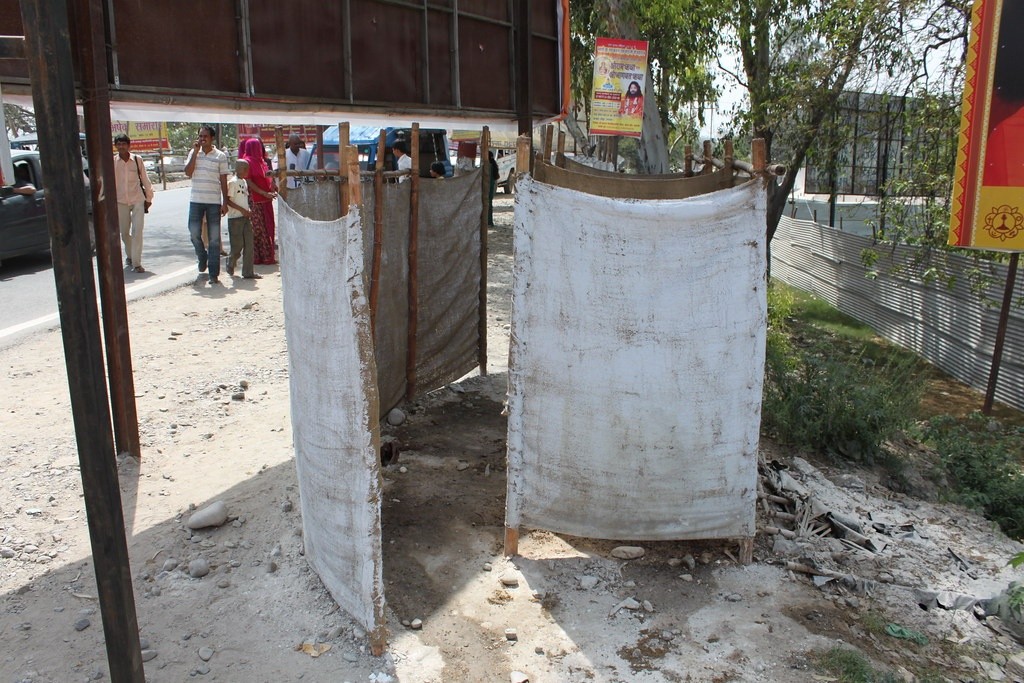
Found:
[430,161,446,179]
[618,81,644,119]
[226,158,263,279]
[114,134,154,273]
[488,151,500,227]
[185,126,230,285]
[273,134,310,189]
[391,139,411,183]
[238,135,278,265]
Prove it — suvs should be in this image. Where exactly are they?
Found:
[9,132,91,178]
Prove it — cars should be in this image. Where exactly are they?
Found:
[0,149,98,261]
[142,156,185,173]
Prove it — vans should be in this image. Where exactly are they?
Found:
[303,124,453,189]
[446,148,517,193]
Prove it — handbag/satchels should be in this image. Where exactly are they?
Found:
[142,189,152,213]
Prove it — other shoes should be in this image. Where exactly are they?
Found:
[226,257,234,275]
[126,258,132,265]
[488,223,493,230]
[244,273,261,278]
[198,251,208,272]
[209,276,217,284]
[220,251,228,256]
[136,266,144,273]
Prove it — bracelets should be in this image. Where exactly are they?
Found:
[262,192,266,196]
